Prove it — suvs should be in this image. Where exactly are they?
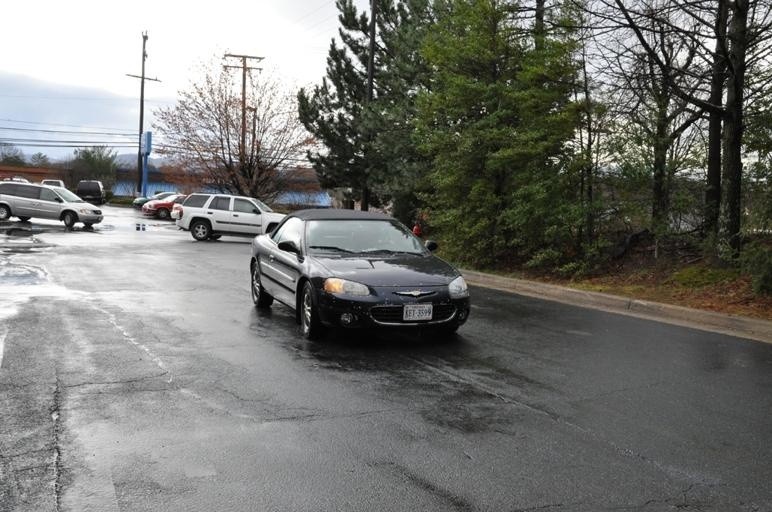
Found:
[170,192,287,241]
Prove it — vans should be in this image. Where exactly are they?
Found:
[0,177,106,227]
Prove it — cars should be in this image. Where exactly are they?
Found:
[250,209,470,340]
[133,192,188,218]
